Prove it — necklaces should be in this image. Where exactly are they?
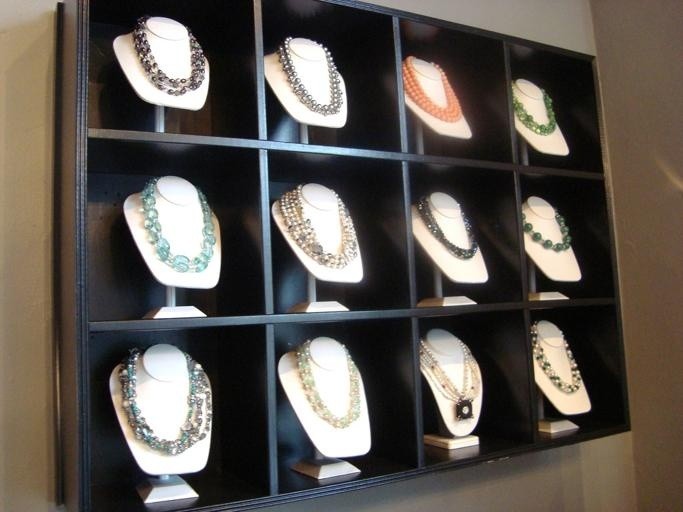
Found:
[133,13,207,96]
[511,79,557,136]
[419,336,482,403]
[401,55,463,122]
[140,175,217,273]
[414,194,478,260]
[522,207,573,252]
[530,320,582,392]
[280,181,359,270]
[295,337,361,429]
[120,344,212,455]
[276,36,344,115]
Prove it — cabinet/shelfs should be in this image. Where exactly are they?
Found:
[54,0,634,512]
